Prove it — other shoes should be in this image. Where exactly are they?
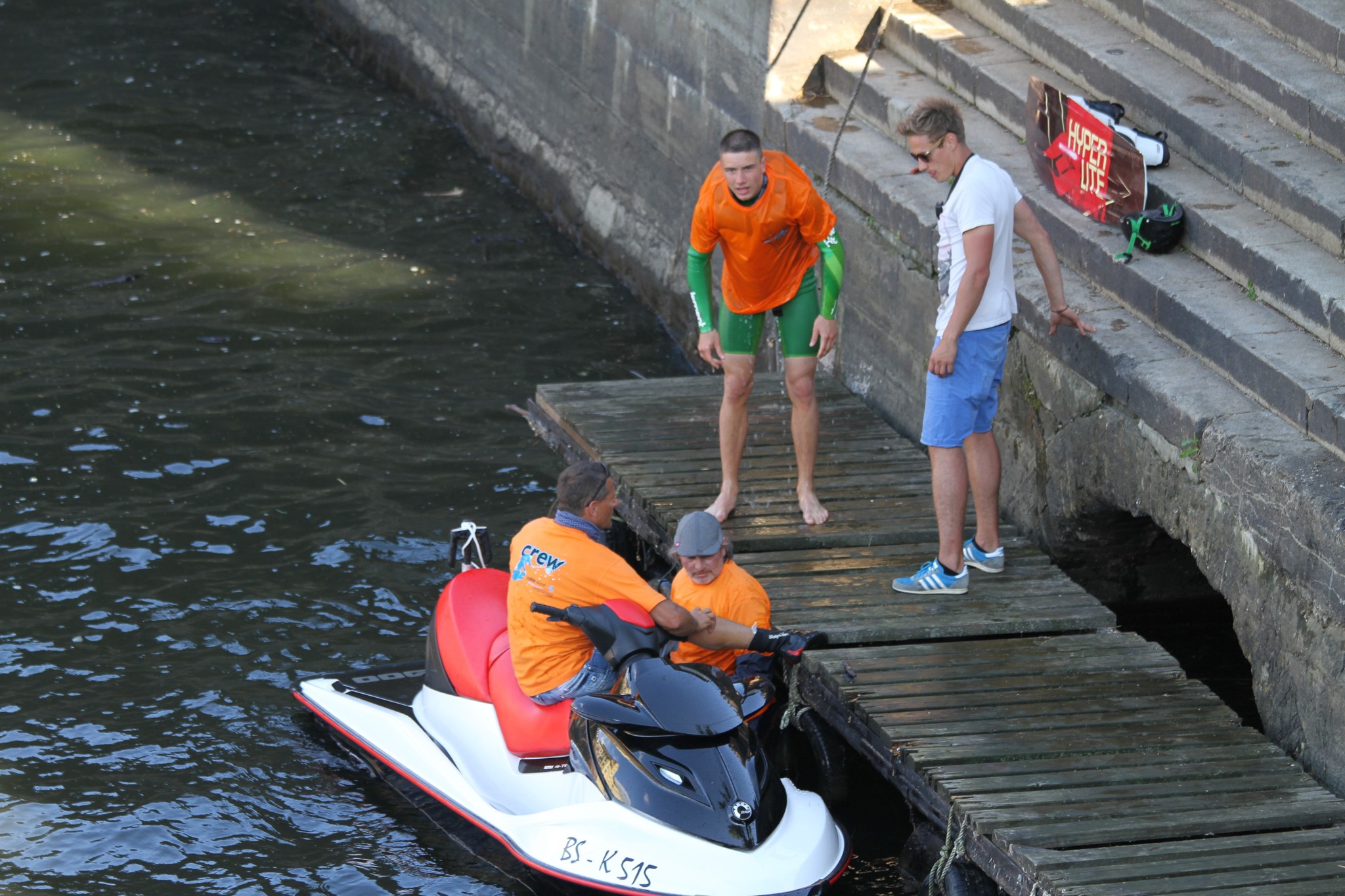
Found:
[1106,124,1171,169]
[1066,95,1125,129]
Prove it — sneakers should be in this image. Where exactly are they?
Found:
[892,559,969,594]
[961,535,1005,573]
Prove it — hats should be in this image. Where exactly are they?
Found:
[673,510,724,557]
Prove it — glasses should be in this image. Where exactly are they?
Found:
[586,460,612,507]
[910,136,945,163]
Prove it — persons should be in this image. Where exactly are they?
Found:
[891,96,1097,594]
[507,463,829,706]
[686,128,845,524]
[670,511,773,683]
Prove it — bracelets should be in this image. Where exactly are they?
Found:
[1052,305,1070,314]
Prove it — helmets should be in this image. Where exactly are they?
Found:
[1120,200,1187,255]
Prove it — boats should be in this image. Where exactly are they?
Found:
[292,520,853,896]
